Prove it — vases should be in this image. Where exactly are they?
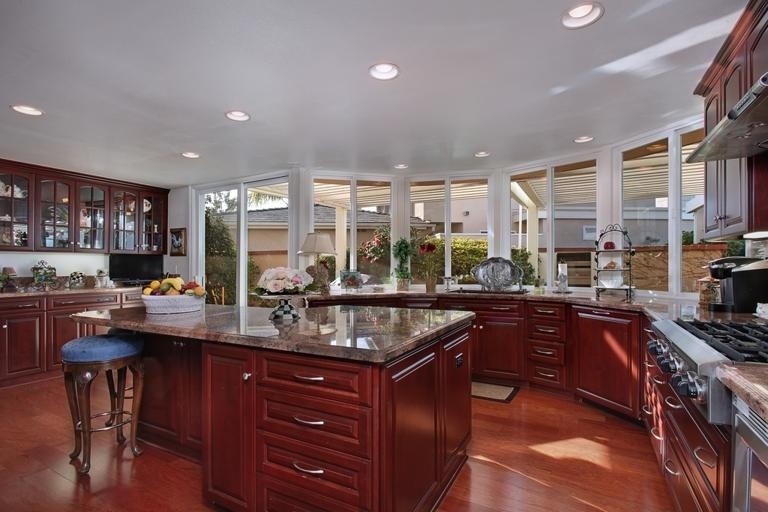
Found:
[425,277,437,293]
[259,294,310,323]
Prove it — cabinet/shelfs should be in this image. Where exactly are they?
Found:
[139,183,173,254]
[202,340,254,512]
[0,295,47,388]
[110,179,141,254]
[47,292,121,382]
[742,0,768,98]
[0,157,34,252]
[641,309,735,512]
[122,290,145,309]
[693,41,767,244]
[526,299,571,394]
[34,165,74,252]
[256,348,381,512]
[402,297,439,310]
[438,296,525,388]
[75,173,109,253]
[438,323,475,483]
[571,305,640,420]
[380,338,440,512]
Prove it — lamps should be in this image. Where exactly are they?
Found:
[367,62,402,82]
[8,105,45,118]
[561,2,607,30]
[391,162,408,171]
[180,152,200,159]
[573,135,596,145]
[224,110,252,123]
[296,233,338,296]
[471,151,491,158]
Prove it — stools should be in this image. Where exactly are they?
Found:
[60,338,147,471]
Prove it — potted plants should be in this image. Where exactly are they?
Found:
[392,238,418,291]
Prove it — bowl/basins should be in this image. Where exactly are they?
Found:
[141,295,205,314]
[600,279,624,288]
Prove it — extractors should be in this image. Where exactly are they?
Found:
[683,71,768,164]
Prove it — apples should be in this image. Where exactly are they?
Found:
[150,287,165,295]
[604,242,616,249]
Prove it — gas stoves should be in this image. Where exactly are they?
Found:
[645,319,768,427]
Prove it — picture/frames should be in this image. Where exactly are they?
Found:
[168,227,187,257]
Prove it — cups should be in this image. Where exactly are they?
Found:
[533,278,545,295]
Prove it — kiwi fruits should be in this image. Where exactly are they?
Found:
[160,283,170,291]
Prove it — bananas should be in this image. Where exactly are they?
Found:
[161,278,181,291]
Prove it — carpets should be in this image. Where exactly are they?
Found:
[472,380,519,404]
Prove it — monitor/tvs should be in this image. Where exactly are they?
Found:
[109,253,164,286]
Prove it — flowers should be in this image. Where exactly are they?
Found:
[419,234,444,278]
[256,265,314,296]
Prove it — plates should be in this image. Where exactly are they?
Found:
[470,256,524,288]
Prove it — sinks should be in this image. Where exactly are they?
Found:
[443,288,533,298]
